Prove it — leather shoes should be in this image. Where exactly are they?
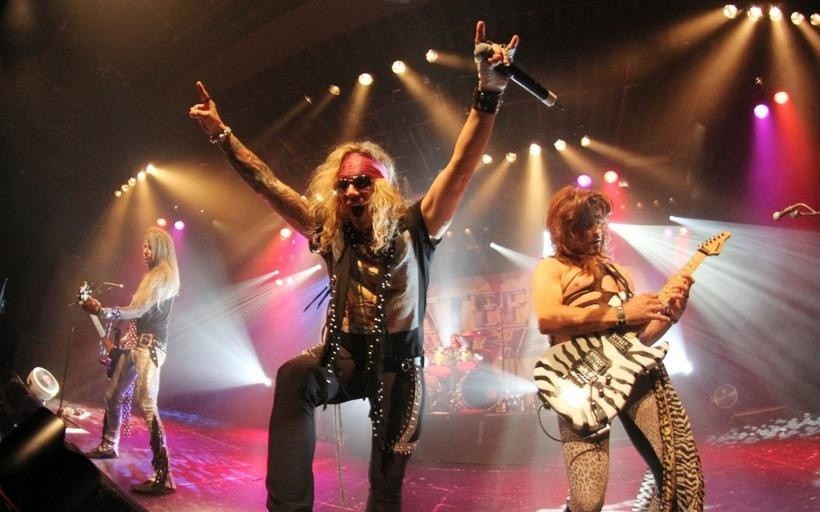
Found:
[87,448,115,458]
[132,481,173,493]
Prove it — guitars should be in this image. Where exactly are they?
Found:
[78,281,122,381]
[533,230,732,432]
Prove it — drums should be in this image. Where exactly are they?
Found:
[424,372,441,410]
[455,348,478,372]
[430,347,454,365]
[457,368,503,411]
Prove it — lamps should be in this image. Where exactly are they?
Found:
[27,366,59,402]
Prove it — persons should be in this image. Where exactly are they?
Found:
[76,227,179,496]
[530,189,706,512]
[188,18,520,510]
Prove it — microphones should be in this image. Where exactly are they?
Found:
[773,203,802,220]
[473,42,557,107]
[101,281,125,289]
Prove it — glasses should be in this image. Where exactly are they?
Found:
[334,174,381,189]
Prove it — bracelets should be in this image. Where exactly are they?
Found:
[97,305,121,320]
[208,124,231,144]
[470,79,504,115]
[613,303,626,328]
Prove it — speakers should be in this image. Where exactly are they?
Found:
[0,370,147,512]
